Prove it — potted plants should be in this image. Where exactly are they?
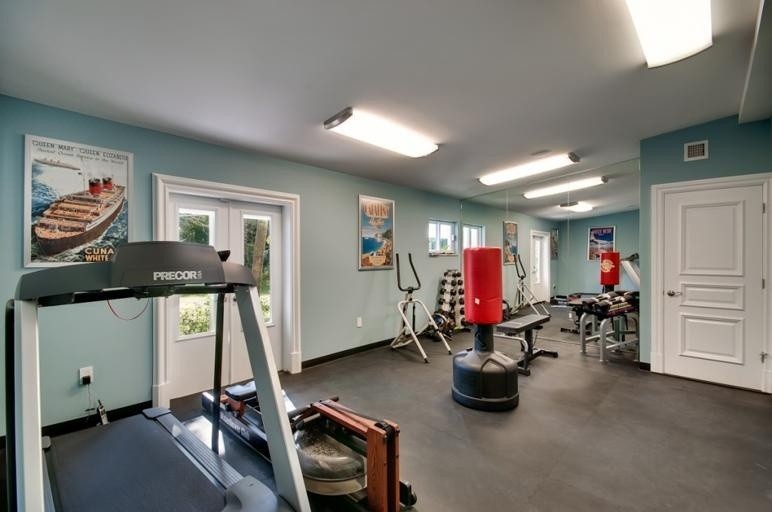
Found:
[586,224,616,264]
[20,131,134,270]
[500,220,520,266]
[357,192,397,273]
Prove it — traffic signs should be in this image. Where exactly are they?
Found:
[79,364,94,385]
